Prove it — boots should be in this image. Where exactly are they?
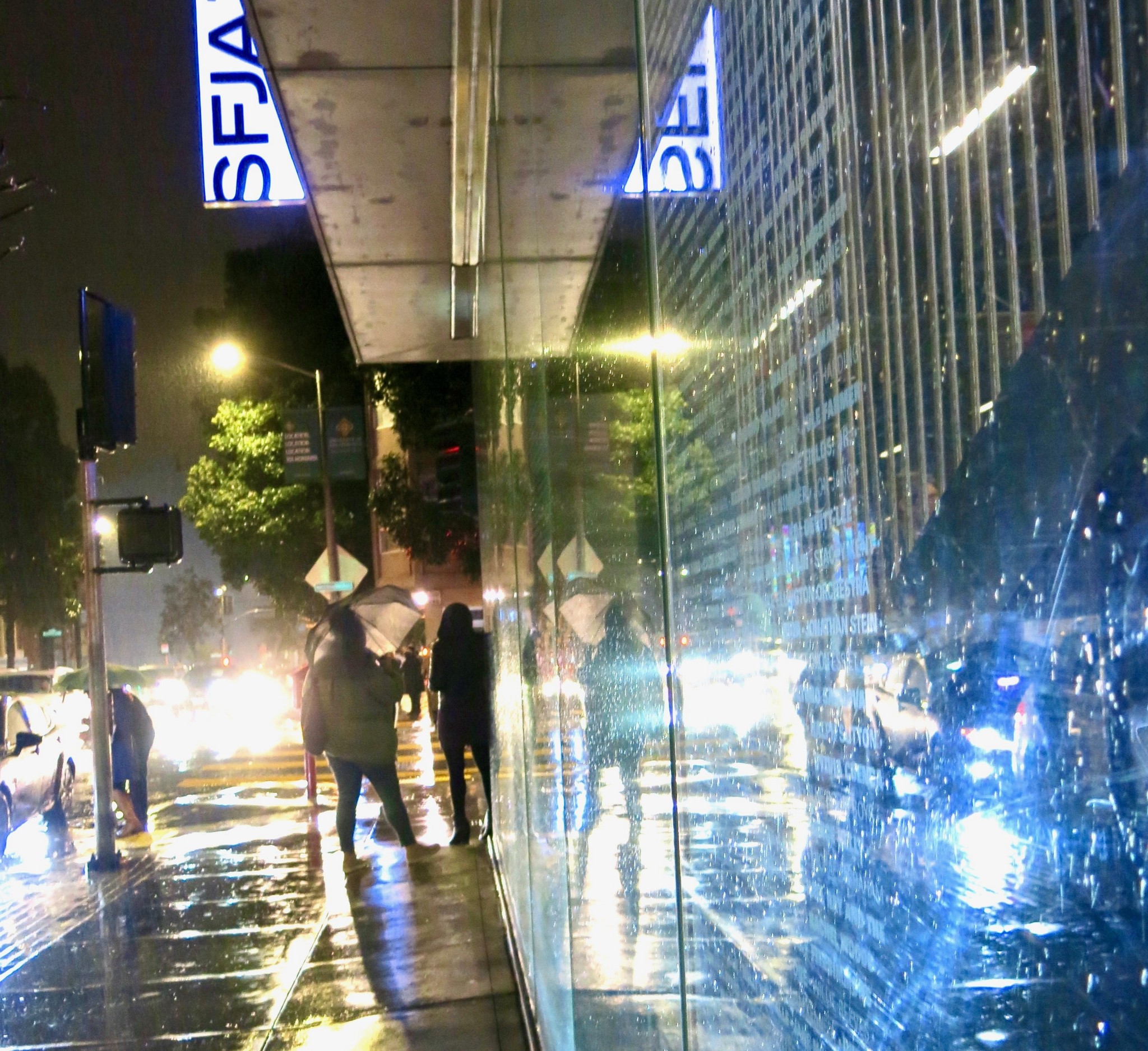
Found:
[112,789,144,838]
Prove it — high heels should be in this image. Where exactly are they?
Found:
[449,819,470,845]
[478,810,493,841]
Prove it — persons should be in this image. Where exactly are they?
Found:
[576,608,663,832]
[427,602,493,846]
[380,644,431,719]
[80,686,155,837]
[301,608,440,870]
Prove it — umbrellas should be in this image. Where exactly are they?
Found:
[49,661,148,692]
[304,586,420,662]
[559,589,656,652]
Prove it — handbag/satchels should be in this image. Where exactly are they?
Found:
[300,681,326,756]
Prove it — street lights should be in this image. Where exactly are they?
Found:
[206,339,341,601]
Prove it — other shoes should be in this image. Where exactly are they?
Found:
[405,841,441,863]
[407,711,421,721]
[343,852,368,874]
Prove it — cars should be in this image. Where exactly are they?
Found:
[146,664,240,706]
[0,696,75,856]
[0,665,152,734]
